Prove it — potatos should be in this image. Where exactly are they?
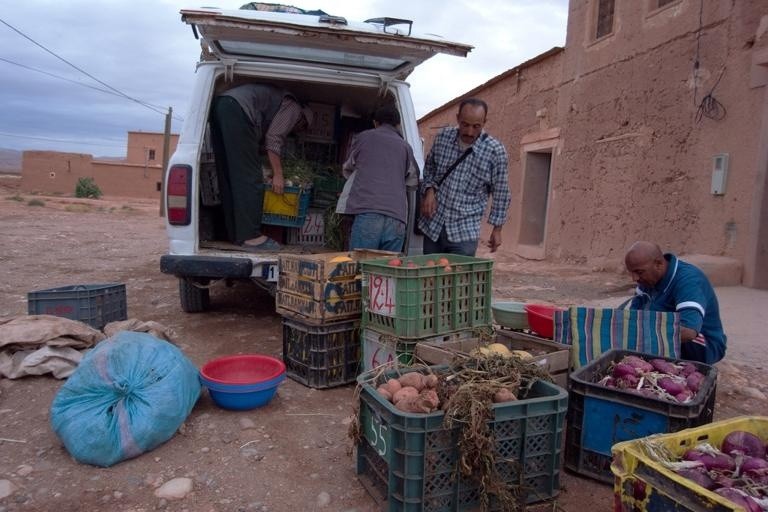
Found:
[375,372,517,413]
[470,342,533,358]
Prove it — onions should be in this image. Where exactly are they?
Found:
[599,355,768,512]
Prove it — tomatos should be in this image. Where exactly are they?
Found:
[388,259,462,326]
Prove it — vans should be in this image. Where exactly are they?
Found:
[161,9,475,314]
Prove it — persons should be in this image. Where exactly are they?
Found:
[623,239,726,365]
[205,80,314,251]
[415,95,512,257]
[334,104,420,253]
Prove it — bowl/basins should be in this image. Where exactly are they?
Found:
[197,352,289,411]
[523,304,571,339]
[491,301,529,330]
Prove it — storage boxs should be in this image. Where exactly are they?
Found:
[201,163,221,206]
[27,283,127,331]
[609,413,768,509]
[360,327,475,373]
[414,323,572,391]
[277,250,361,327]
[277,313,363,393]
[564,347,719,491]
[357,251,496,340]
[349,359,571,509]
[262,104,345,247]
